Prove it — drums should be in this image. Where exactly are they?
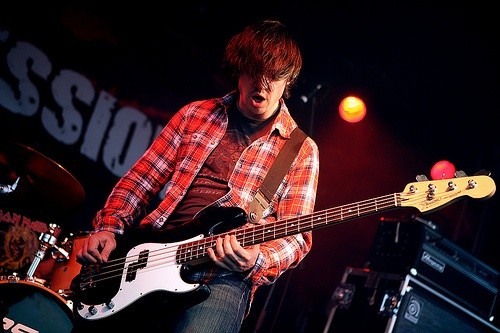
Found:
[51,231,92,294]
[0,272,74,333]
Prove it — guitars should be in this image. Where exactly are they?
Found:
[71,168,496,331]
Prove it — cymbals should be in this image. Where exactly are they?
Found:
[0,137,89,209]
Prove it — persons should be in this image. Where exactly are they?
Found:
[75,20,319,333]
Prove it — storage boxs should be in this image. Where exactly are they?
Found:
[325,266,500,333]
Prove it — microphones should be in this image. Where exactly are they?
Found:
[301,85,321,103]
